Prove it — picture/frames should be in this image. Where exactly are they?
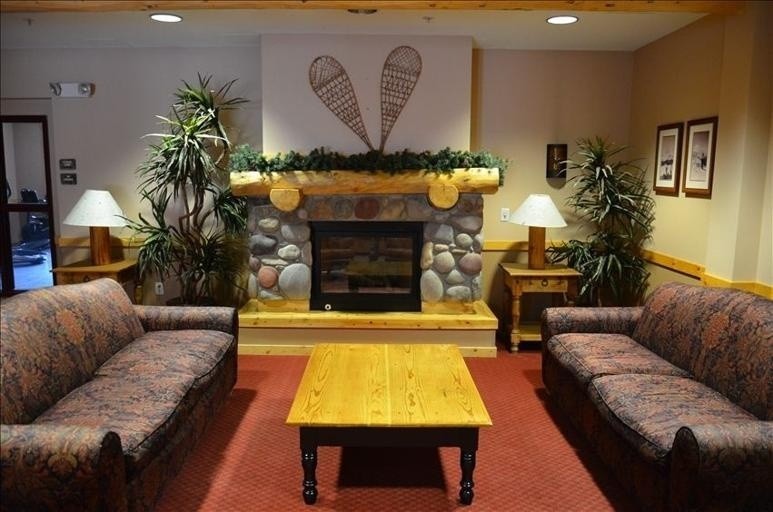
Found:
[653,122,684,193]
[682,115,718,194]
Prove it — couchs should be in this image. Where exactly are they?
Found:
[0,277,239,511]
[540,282,772,512]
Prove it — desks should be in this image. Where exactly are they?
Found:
[49,258,139,306]
[499,262,583,354]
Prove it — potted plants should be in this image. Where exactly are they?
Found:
[556,136,655,307]
[113,72,250,307]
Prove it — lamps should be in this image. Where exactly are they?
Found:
[63,189,128,265]
[509,193,567,269]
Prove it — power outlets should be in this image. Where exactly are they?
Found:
[154,282,164,296]
[500,208,510,223]
